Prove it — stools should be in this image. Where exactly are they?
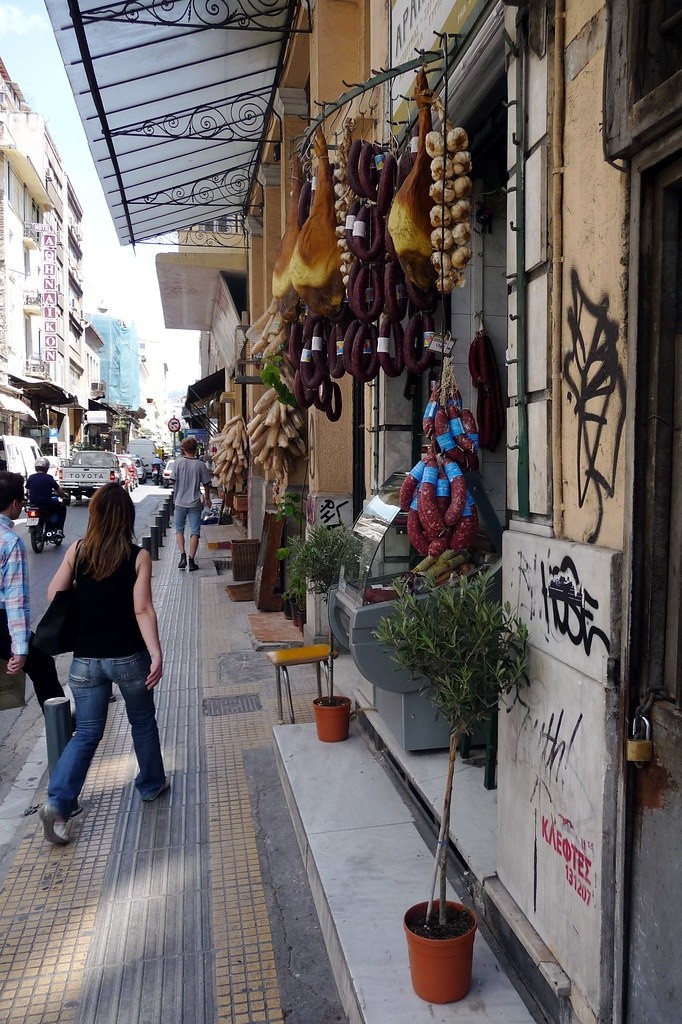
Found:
[267,646,339,724]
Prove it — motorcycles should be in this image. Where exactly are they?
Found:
[24,490,69,553]
[151,463,165,486]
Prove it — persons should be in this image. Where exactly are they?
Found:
[39,482,172,846]
[168,437,212,571]
[0,469,66,714]
[25,458,66,538]
[149,453,166,482]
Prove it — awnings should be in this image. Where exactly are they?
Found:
[0,379,120,422]
[43,0,303,248]
[182,367,226,418]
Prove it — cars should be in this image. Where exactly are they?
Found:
[22,490,71,507]
[43,456,60,481]
[96,455,149,492]
[162,460,176,488]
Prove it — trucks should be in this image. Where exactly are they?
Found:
[0,435,44,495]
[126,439,156,475]
[59,451,126,500]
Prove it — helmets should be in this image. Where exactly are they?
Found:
[35,458,49,472]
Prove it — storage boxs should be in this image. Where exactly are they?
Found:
[229,537,261,581]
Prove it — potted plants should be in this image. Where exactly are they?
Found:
[274,524,367,742]
[375,573,532,1006]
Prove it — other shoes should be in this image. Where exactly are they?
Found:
[178,553,187,568]
[189,559,199,571]
[57,530,65,537]
[72,711,77,734]
[143,777,169,800]
[39,805,71,844]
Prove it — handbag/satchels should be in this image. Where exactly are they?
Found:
[32,539,85,655]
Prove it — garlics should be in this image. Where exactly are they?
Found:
[333,154,351,297]
[425,127,472,293]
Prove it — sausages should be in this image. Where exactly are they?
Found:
[469,334,506,454]
[345,139,439,380]
[286,164,345,423]
[400,381,479,557]
